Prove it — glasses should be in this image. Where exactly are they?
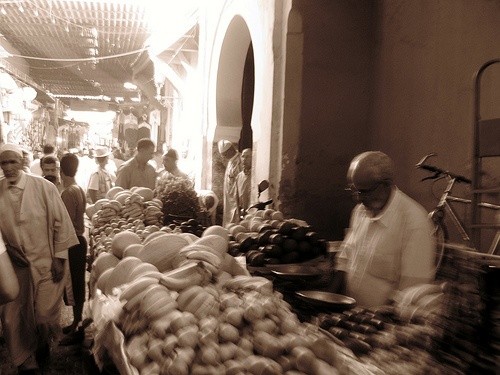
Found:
[343,185,379,196]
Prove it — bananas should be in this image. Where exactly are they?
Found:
[111,245,273,336]
[93,200,164,225]
[394,283,449,324]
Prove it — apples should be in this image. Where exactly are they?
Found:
[124,289,354,375]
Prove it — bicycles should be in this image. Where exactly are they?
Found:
[415,152,500,279]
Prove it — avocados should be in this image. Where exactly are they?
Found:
[230,222,327,266]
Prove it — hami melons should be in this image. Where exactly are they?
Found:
[92,224,246,293]
[86,187,154,219]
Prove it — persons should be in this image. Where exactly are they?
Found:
[88,148,116,204]
[0,143,88,375]
[218,139,252,228]
[328,152,437,308]
[155,149,195,190]
[115,138,157,191]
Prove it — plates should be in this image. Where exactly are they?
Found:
[294,290,356,309]
[270,264,324,280]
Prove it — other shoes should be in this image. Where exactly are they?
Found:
[59,332,85,344]
[62,325,75,333]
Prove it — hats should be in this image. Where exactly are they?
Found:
[92,147,111,158]
[218,140,232,153]
[0,143,22,158]
